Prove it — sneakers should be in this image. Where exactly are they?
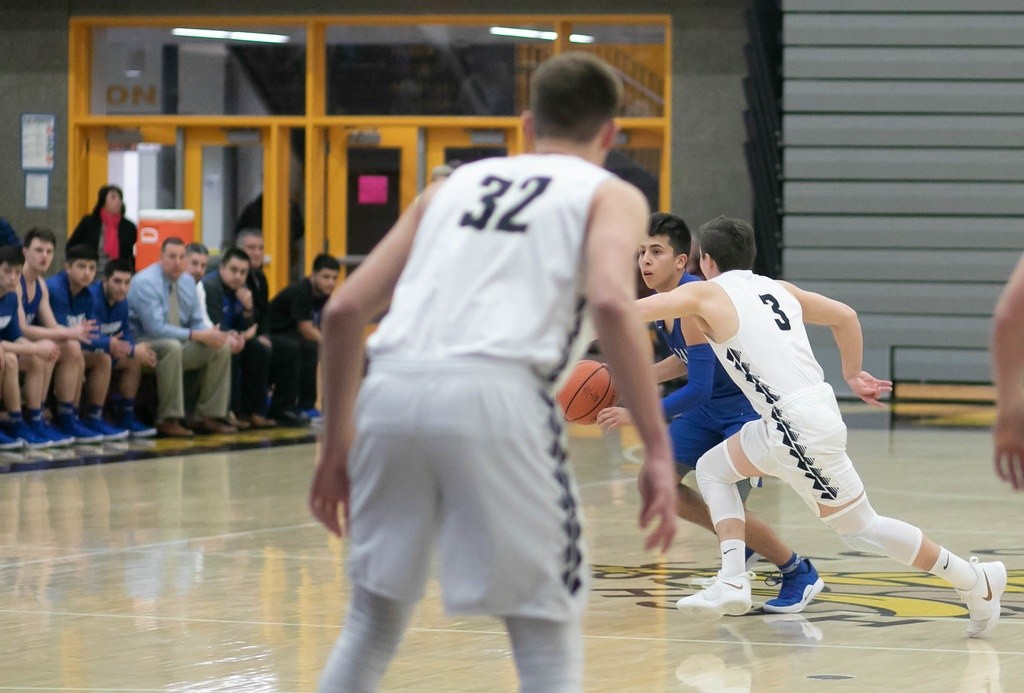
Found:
[676,654,752,693]
[763,615,823,655]
[716,623,759,670]
[32,422,76,447]
[86,417,130,440]
[120,416,158,437]
[0,408,24,449]
[5,420,53,449]
[186,420,236,434]
[955,555,1008,639]
[743,546,761,571]
[245,414,276,429]
[677,571,756,620]
[0,438,156,462]
[57,419,104,444]
[962,640,1003,693]
[224,412,250,430]
[761,555,823,613]
[151,419,194,437]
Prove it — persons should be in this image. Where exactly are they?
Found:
[206,228,307,429]
[66,185,138,273]
[0,215,157,451]
[308,53,679,692]
[637,212,825,614]
[589,216,1007,638]
[233,170,304,280]
[990,255,1024,494]
[126,237,238,437]
[203,246,277,428]
[268,253,341,410]
[184,242,245,353]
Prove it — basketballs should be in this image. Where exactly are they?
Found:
[555,359,618,425]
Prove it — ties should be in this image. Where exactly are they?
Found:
[169,282,181,327]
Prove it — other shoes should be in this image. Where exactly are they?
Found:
[276,411,310,426]
[302,409,322,419]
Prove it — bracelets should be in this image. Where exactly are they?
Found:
[189,328,192,341]
